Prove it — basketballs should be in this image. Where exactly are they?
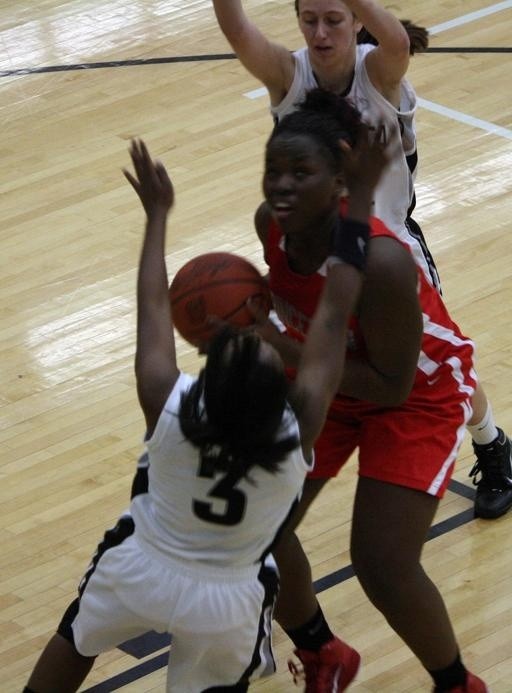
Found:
[169,252,269,352]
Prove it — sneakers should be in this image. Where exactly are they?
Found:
[294,638,360,692]
[469,427,511,521]
[431,671,487,693]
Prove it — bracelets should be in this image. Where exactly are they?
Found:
[335,216,371,272]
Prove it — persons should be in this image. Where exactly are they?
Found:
[198,84,493,691]
[18,107,412,693]
[212,0,511,525]
